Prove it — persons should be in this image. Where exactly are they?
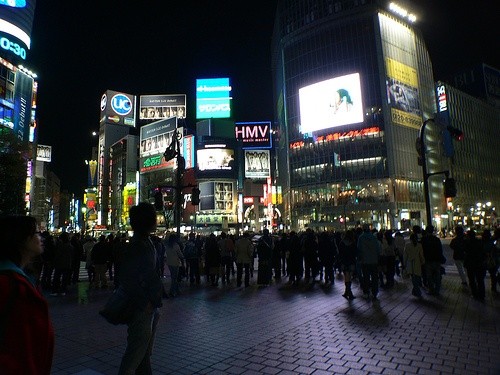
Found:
[0,214,55,374]
[25,232,126,296]
[328,90,353,117]
[114,203,163,374]
[150,225,500,301]
[246,151,269,172]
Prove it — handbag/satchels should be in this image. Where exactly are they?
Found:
[99,277,143,324]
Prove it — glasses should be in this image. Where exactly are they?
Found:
[33,230,43,236]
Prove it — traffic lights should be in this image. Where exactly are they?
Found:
[154,192,163,211]
[448,125,463,141]
[192,188,201,205]
